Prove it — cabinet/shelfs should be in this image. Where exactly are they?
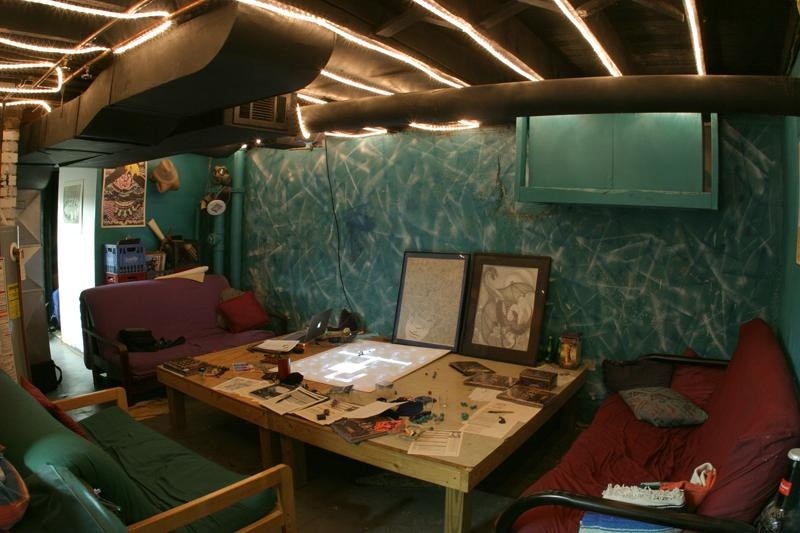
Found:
[514,112,718,209]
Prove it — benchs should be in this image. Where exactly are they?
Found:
[0,368,294,533]
[492,318,800,533]
[78,275,290,406]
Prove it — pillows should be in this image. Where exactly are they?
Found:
[616,385,709,427]
[216,287,272,335]
[671,347,725,412]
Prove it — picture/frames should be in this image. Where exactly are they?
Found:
[459,253,551,368]
[391,251,471,353]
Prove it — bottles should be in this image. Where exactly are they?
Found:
[754,448,800,533]
[545,336,554,363]
[556,337,561,364]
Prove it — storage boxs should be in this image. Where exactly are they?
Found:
[103,244,166,285]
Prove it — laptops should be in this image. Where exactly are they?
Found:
[280,308,332,343]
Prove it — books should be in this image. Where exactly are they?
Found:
[465,372,519,391]
[449,361,495,377]
[519,369,557,391]
[331,414,396,443]
[497,384,557,408]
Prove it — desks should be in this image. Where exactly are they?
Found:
[158,327,589,533]
